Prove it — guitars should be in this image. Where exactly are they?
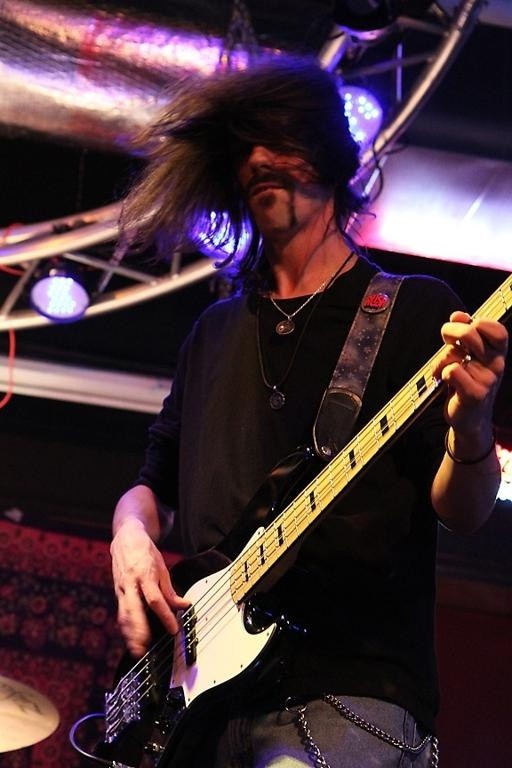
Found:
[105,274,512,767]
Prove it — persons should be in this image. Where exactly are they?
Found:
[105,49,509,766]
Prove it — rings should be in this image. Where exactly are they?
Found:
[460,355,471,370]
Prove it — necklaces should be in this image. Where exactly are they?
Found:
[255,249,355,410]
[266,252,356,338]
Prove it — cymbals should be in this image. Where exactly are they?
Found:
[0,675,60,753]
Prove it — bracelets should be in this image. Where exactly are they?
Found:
[442,424,497,467]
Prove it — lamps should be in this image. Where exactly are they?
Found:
[30,264,92,323]
